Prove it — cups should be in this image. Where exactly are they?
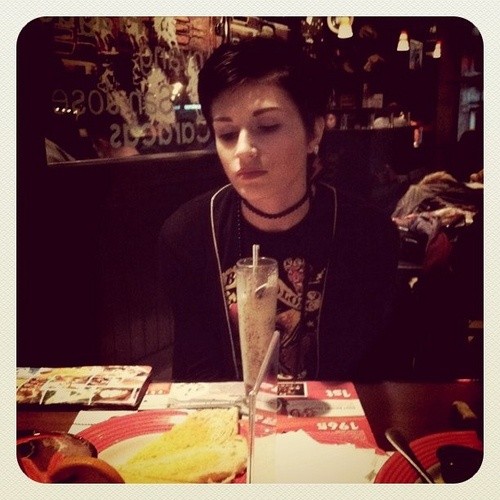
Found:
[231,258,279,425]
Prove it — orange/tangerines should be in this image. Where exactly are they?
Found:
[49,456,125,484]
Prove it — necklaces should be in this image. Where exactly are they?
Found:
[240,187,312,220]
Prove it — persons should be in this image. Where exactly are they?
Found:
[160,34,398,383]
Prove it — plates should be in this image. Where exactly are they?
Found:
[66,406,254,483]
[375,430,482,484]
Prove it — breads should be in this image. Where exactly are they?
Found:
[118,436,247,484]
[122,407,239,465]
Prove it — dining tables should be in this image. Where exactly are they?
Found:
[15,378,484,484]
